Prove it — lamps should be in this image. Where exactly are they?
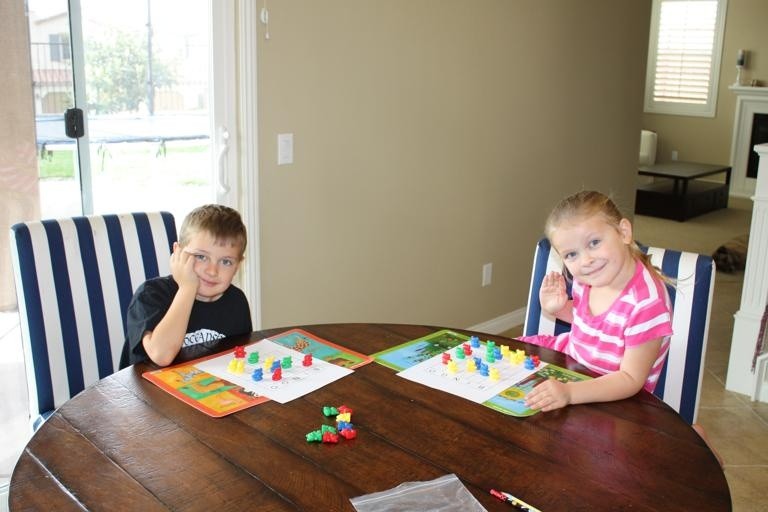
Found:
[733,49,747,86]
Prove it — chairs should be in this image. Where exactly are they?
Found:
[7,210,178,432]
[638,129,658,187]
[521,236,717,425]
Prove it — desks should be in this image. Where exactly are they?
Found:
[8,321,733,511]
[634,160,732,222]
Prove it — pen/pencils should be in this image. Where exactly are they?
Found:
[488,488,537,512]
[500,491,543,512]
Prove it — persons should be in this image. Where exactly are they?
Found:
[510,189,674,414]
[117,203,254,375]
